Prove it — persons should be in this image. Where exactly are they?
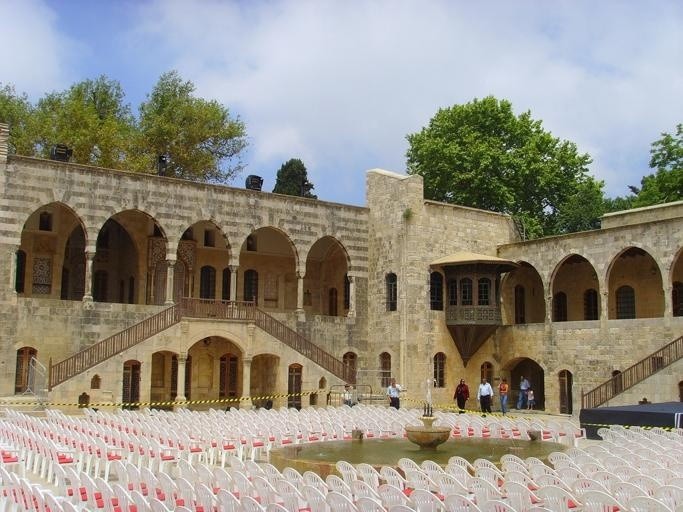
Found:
[341,384,352,408]
[526,387,536,410]
[517,375,530,410]
[477,377,494,413]
[497,377,509,415]
[387,378,402,410]
[454,379,469,415]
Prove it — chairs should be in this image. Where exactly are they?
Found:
[0,404,681,512]
